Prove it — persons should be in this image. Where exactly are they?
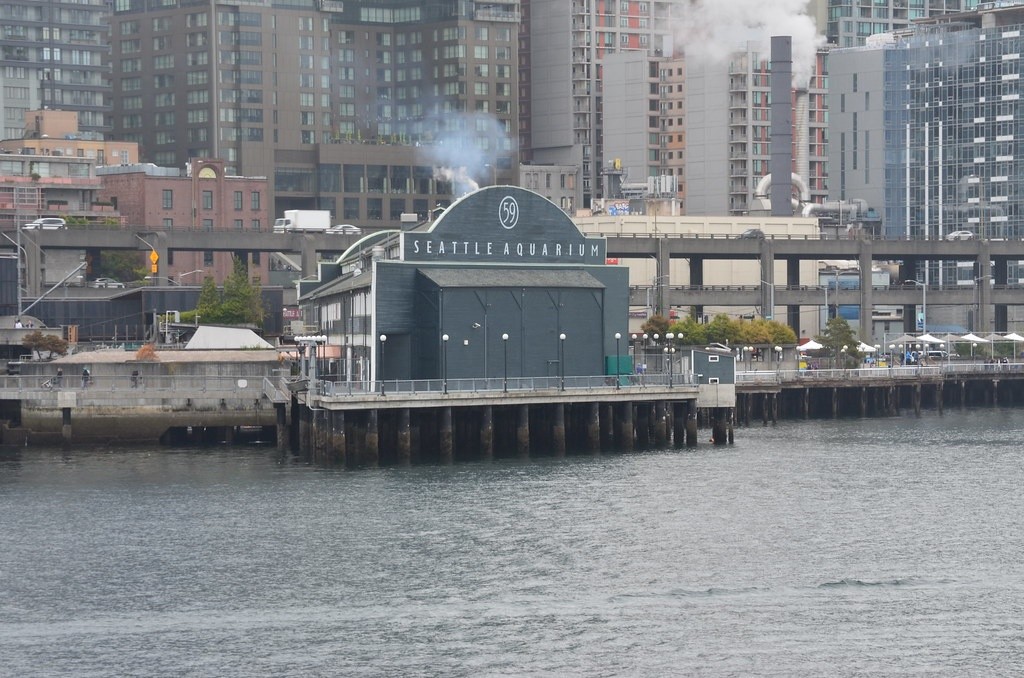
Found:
[14,319,24,328]
[900,350,936,367]
[26,320,34,329]
[806,364,811,370]
[814,361,817,369]
[636,363,643,386]
[984,355,1008,370]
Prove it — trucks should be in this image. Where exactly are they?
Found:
[272,210,331,234]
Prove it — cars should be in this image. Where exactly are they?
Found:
[326,224,362,235]
[735,229,765,240]
[946,231,975,241]
[93,277,125,288]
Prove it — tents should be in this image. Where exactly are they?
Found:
[841,340,876,369]
[795,340,829,369]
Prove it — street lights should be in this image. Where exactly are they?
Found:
[484,164,497,186]
[559,333,566,391]
[973,275,994,361]
[841,345,848,380]
[379,334,387,396]
[861,343,865,369]
[774,346,782,368]
[874,345,880,367]
[973,343,977,364]
[668,332,674,388]
[889,344,945,377]
[442,334,450,394]
[796,345,801,381]
[144,269,205,286]
[744,346,753,373]
[294,335,327,395]
[632,333,684,374]
[905,279,926,357]
[502,333,509,393]
[646,275,669,322]
[615,332,621,390]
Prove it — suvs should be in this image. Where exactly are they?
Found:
[22,218,68,230]
